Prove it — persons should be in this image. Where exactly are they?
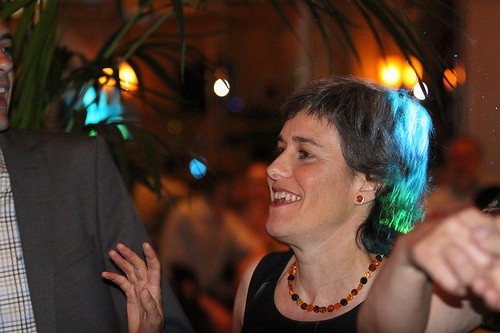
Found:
[101,75,433,333]
[356,182,500,333]
[0,13,194,333]
[134,131,499,333]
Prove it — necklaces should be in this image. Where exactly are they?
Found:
[287,253,386,313]
